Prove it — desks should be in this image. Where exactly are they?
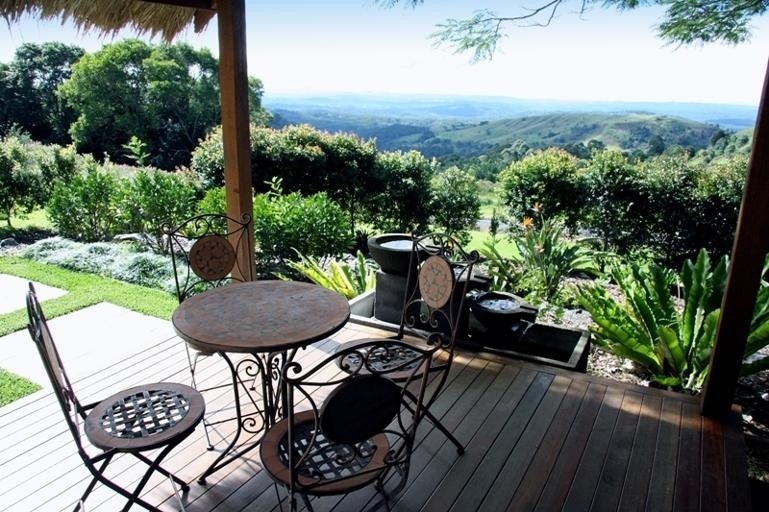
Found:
[172,282,351,511]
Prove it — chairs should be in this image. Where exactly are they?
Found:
[257,329,447,511]
[23,280,206,512]
[161,210,284,452]
[335,228,480,488]
